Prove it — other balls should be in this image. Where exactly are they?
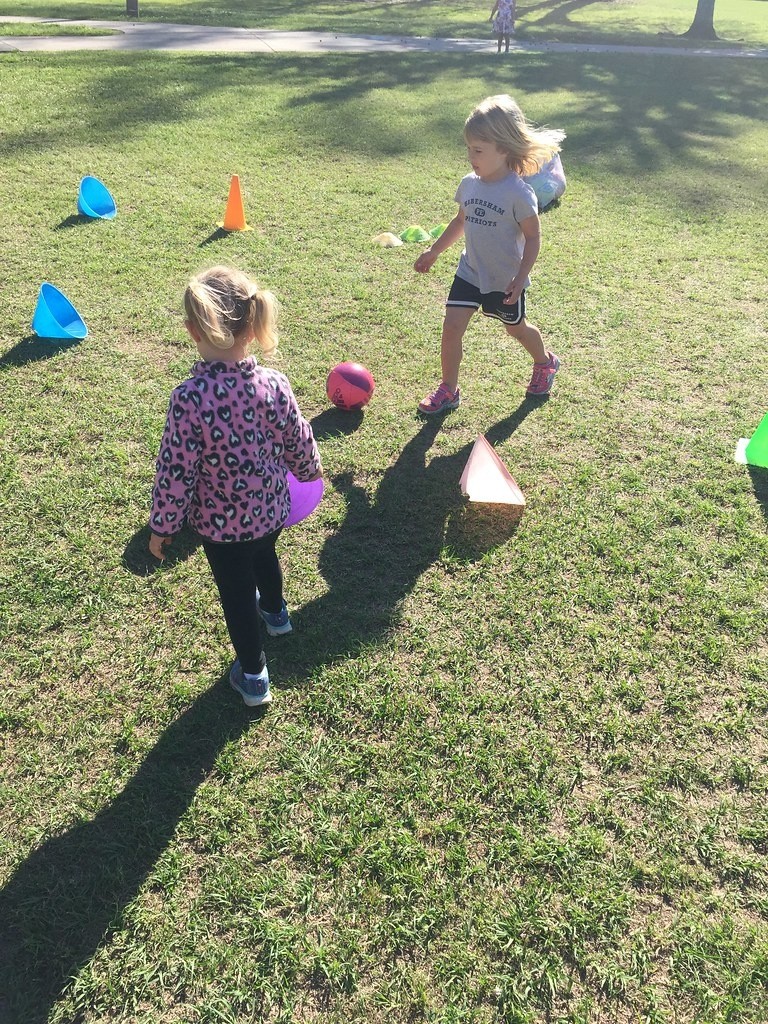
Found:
[327,363,375,411]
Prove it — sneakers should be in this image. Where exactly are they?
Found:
[418,384,460,414]
[255,586,292,636]
[527,351,560,395]
[229,658,272,706]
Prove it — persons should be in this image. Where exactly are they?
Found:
[149,266,324,709]
[413,92,567,415]
[489,0,517,54]
